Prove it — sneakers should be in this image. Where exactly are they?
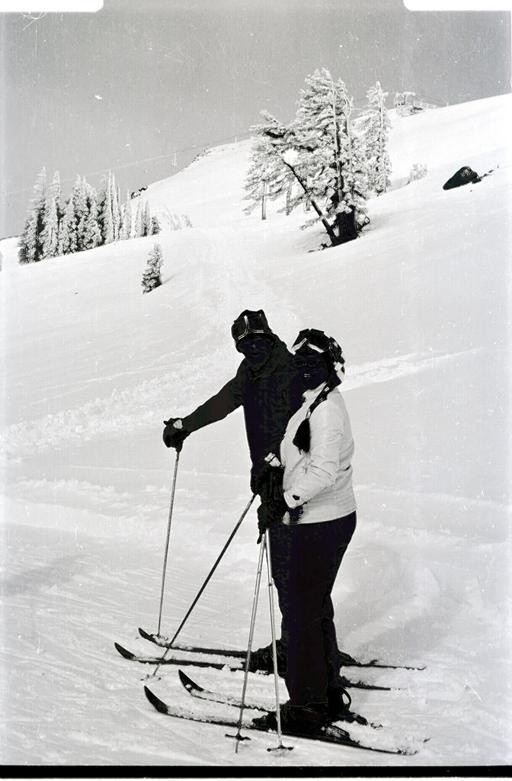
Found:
[326,687,355,721]
[253,703,332,736]
[250,639,354,674]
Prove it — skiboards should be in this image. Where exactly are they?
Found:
[143,669,430,755]
[114,627,427,690]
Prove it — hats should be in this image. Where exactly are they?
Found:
[294,329,343,363]
[231,310,274,352]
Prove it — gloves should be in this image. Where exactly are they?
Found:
[164,419,188,452]
[250,462,271,486]
[260,500,284,527]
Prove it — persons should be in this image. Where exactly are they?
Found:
[163,308,351,672]
[251,328,357,734]
